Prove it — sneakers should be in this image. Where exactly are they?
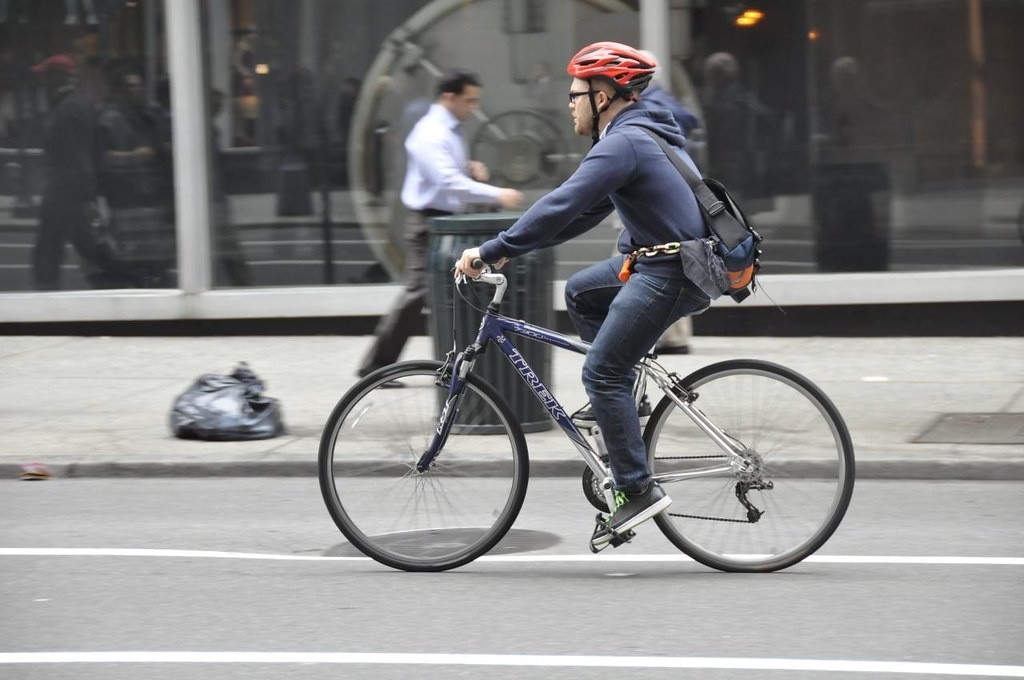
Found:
[592,479,672,544]
[572,394,651,428]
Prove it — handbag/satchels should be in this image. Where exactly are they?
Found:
[694,178,764,303]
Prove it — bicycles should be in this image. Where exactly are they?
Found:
[318,260,858,574]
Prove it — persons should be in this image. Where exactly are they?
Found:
[455,42,764,545]
[529,57,562,179]
[139,87,251,287]
[614,49,693,356]
[699,51,748,201]
[356,68,522,387]
[28,56,135,291]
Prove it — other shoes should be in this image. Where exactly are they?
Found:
[380,382,404,389]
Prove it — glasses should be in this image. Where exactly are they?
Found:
[570,90,610,103]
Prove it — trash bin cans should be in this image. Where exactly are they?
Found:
[810,163,894,272]
[424,211,556,434]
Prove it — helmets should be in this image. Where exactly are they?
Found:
[567,42,656,100]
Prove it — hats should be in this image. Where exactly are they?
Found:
[32,56,75,74]
